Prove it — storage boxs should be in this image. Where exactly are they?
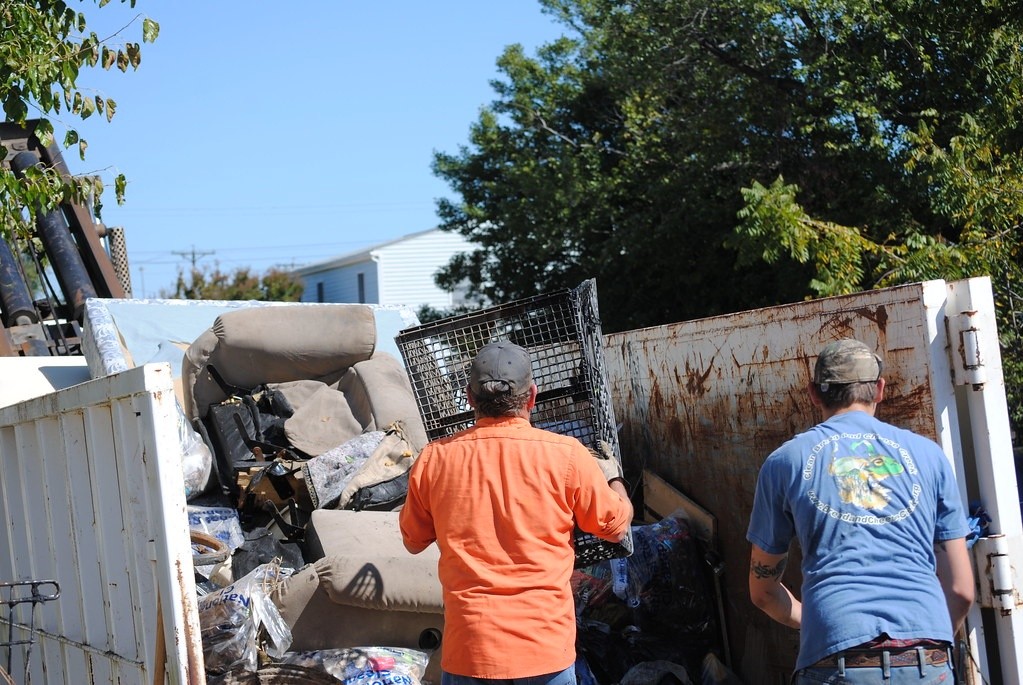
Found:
[394,278,634,571]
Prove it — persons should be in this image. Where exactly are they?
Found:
[746,338,978,685]
[397,339,635,685]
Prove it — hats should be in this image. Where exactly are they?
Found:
[470,340,533,397]
[813,338,883,383]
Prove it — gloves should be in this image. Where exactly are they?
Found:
[589,440,627,485]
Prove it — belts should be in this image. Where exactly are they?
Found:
[810,646,949,670]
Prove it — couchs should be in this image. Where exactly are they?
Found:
[180,305,445,653]
